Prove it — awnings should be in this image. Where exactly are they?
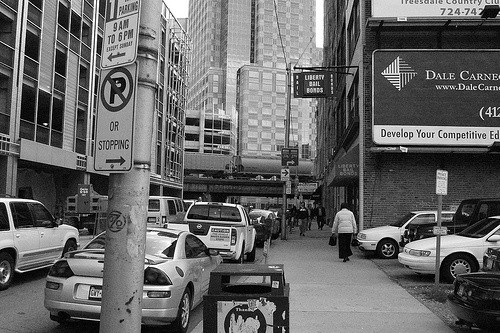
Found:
[327,164,359,188]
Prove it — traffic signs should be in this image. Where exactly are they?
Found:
[98,0,142,71]
[281,148,298,168]
[280,168,290,181]
[93,60,139,173]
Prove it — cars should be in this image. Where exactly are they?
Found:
[248,207,282,245]
[398,215,500,283]
[443,247,500,331]
[44,228,223,333]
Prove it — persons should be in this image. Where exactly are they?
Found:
[328,203,360,263]
[297,202,308,237]
[316,202,326,230]
[306,203,314,231]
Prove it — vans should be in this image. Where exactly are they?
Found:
[147,196,199,227]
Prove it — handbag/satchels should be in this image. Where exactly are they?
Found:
[329,234,337,246]
[351,234,360,246]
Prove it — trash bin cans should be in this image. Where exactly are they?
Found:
[202,263,290,333]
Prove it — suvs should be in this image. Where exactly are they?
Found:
[399,199,500,247]
[357,211,470,259]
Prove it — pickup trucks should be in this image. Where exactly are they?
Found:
[0,198,80,291]
[163,201,256,264]
